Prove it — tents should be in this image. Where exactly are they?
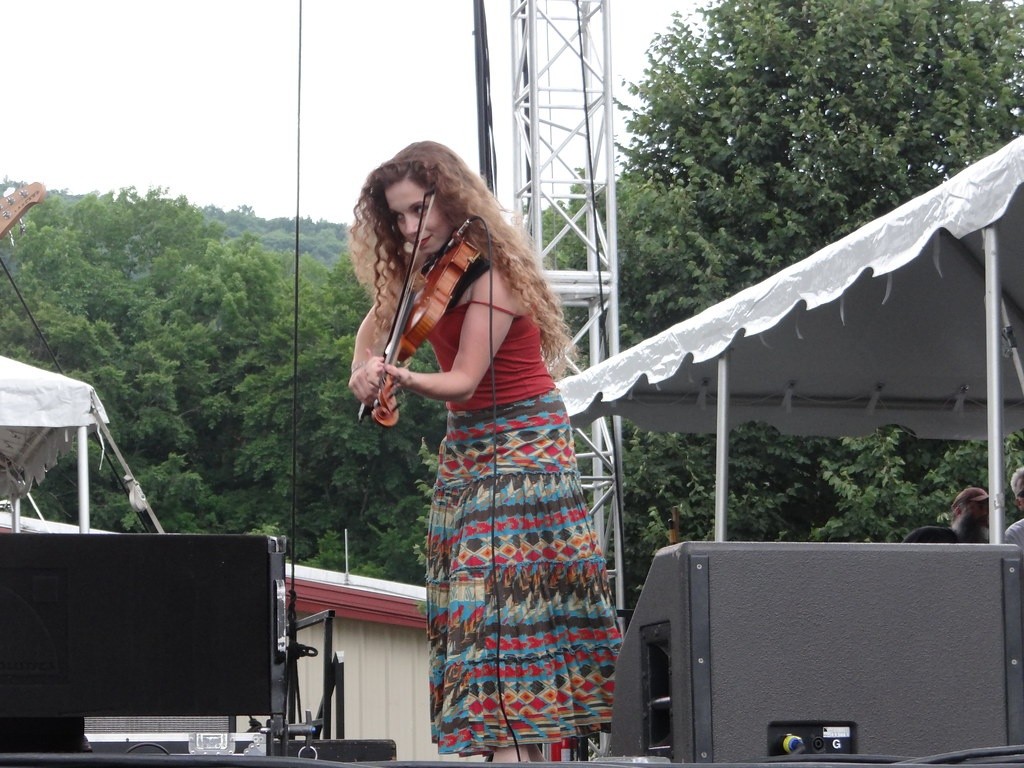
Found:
[559,130,1024,543]
[0,355,110,534]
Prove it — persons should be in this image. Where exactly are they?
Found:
[347,141,624,761]
[1004,466,1024,544]
[904,488,989,543]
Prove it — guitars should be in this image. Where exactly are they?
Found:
[1,181,47,248]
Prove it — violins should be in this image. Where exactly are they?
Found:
[370,229,481,427]
